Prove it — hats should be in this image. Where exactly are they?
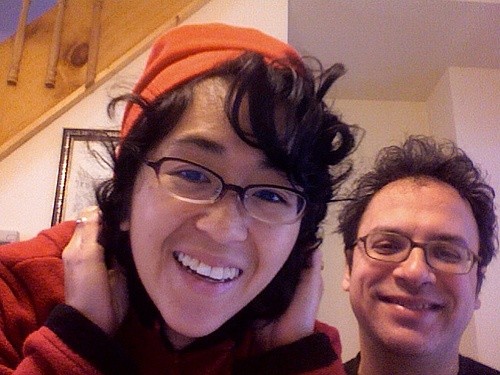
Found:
[121,22,304,144]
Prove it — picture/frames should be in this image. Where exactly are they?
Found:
[51,128,121,232]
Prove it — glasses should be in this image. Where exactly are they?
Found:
[139,156,308,226]
[353,230,480,276]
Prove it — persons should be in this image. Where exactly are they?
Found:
[0,23,366,375]
[330,134,500,375]
